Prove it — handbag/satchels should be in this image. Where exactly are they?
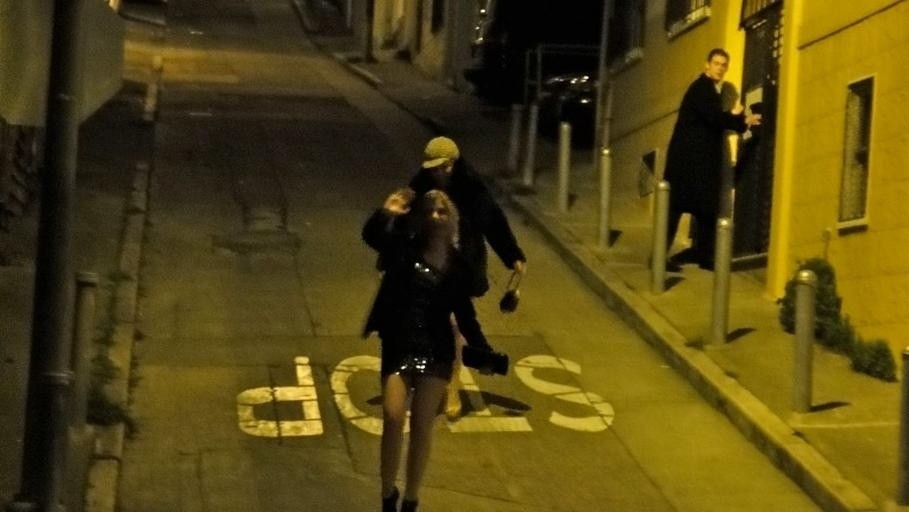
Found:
[499,269,523,314]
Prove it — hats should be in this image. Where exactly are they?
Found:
[422,136,461,169]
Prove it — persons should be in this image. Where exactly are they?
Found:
[648,48,763,274]
[409,134,525,421]
[359,186,493,512]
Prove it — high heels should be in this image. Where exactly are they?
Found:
[382,486,419,512]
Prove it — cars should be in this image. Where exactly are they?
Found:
[538,42,615,150]
